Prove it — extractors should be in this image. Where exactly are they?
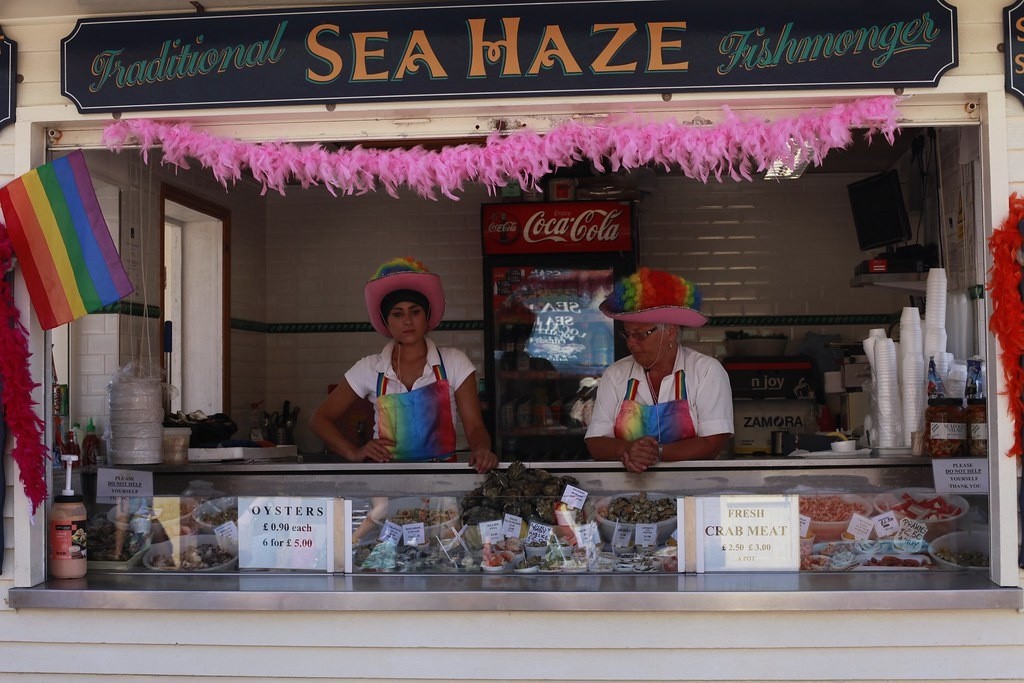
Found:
[848,242,942,293]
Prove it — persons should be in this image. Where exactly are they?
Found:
[584,269,735,473]
[306,258,499,475]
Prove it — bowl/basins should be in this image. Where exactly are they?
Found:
[92,490,990,576]
[726,335,787,357]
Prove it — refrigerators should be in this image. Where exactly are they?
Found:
[478,199,643,463]
[838,391,872,432]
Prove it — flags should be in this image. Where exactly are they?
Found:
[0,149,134,330]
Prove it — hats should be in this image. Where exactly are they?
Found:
[599,268,709,329]
[364,259,446,338]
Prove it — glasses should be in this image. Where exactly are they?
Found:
[617,326,662,340]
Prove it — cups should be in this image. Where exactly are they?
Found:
[858,267,987,458]
[832,440,856,454]
[162,427,192,466]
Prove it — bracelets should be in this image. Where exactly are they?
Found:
[657,445,664,462]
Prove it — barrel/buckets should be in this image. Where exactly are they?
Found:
[770,430,790,456]
[823,370,847,395]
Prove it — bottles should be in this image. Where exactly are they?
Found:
[65,417,107,466]
[247,400,265,430]
[501,321,612,371]
[806,404,816,432]
[502,439,518,461]
[818,405,836,432]
[48,494,88,580]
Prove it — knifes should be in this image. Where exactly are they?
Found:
[287,405,301,428]
[281,400,290,427]
